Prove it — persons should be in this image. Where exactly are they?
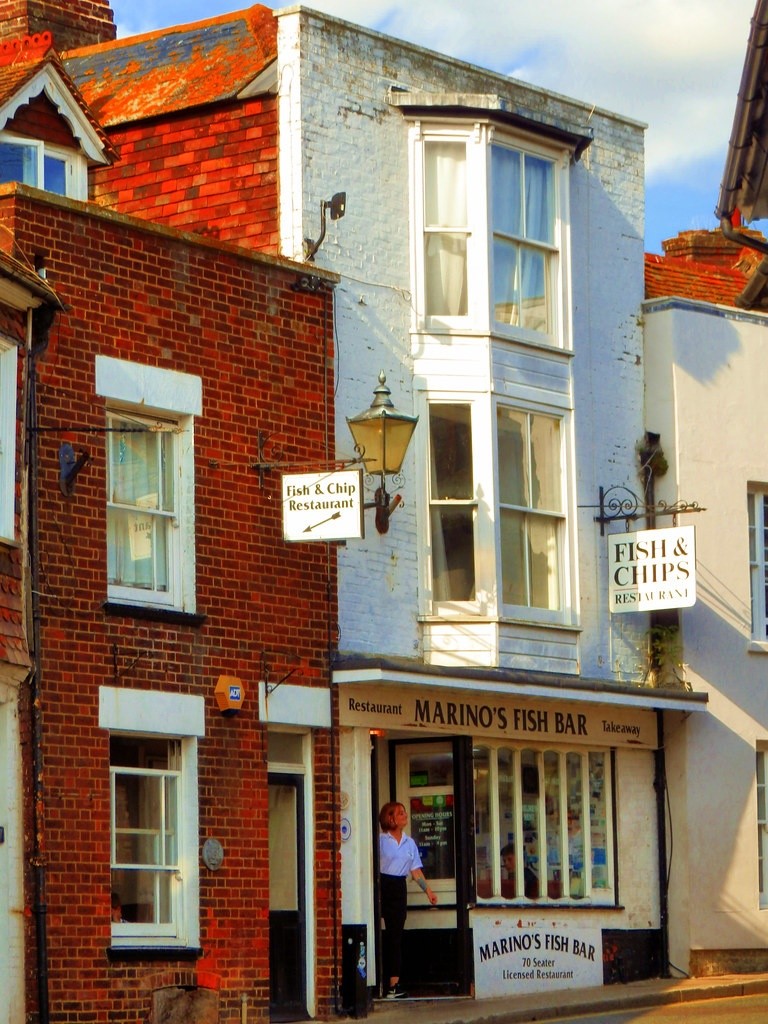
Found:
[376,802,438,998]
[500,843,542,900]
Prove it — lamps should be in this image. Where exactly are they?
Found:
[249,371,420,533]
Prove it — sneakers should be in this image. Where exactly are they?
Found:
[386,982,409,999]
